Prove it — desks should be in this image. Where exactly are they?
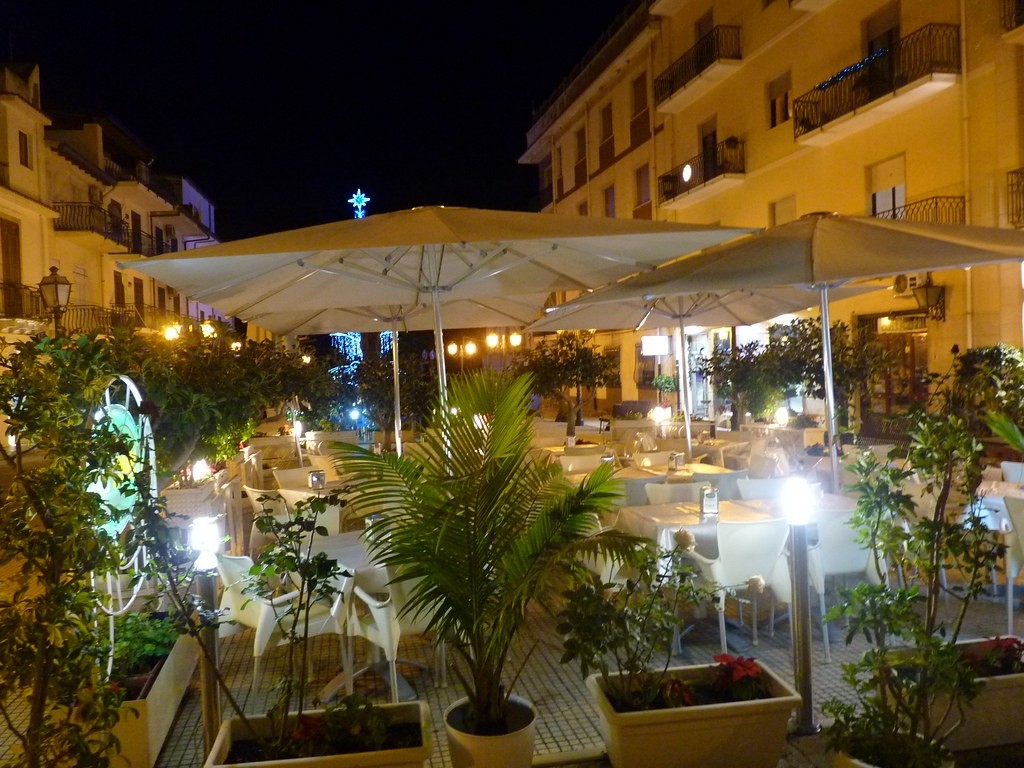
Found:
[249,449,265,489]
[622,494,885,645]
[279,479,424,540]
[768,426,828,449]
[640,463,739,486]
[222,475,245,556]
[241,458,252,488]
[541,443,599,456]
[281,528,494,665]
[565,467,667,506]
[691,441,751,467]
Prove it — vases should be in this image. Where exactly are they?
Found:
[281,432,286,436]
[865,634,1024,751]
[582,661,802,768]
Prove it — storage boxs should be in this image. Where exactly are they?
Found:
[308,469,325,489]
[668,453,685,470]
[600,455,615,472]
[697,431,709,444]
[566,436,575,448]
[700,486,720,514]
[365,515,393,543]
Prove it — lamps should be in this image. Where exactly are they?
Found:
[913,285,947,321]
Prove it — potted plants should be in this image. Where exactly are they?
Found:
[236,420,256,450]
[49,610,200,768]
[157,439,216,503]
[133,473,435,768]
[826,412,1007,768]
[333,377,653,768]
[204,428,236,474]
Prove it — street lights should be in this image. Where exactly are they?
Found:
[448,342,475,378]
[36,266,74,340]
[487,333,522,372]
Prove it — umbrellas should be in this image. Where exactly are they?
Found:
[117,197,1024,499]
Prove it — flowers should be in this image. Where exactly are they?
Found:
[277,425,289,432]
[559,527,762,710]
[985,631,1024,672]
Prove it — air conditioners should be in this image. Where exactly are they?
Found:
[166,225,175,237]
[91,188,103,202]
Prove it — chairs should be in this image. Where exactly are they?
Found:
[214,408,1024,702]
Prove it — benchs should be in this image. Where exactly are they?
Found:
[598,399,650,434]
